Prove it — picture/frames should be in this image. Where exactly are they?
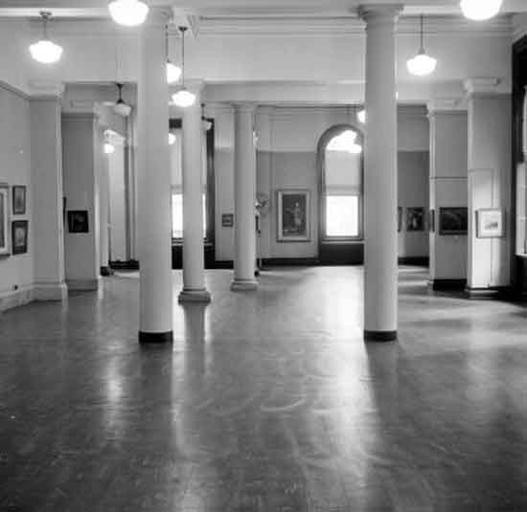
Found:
[276,188,311,242]
[476,208,505,238]
[1,183,29,256]
[438,206,469,235]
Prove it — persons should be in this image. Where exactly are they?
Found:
[290,201,302,226]
